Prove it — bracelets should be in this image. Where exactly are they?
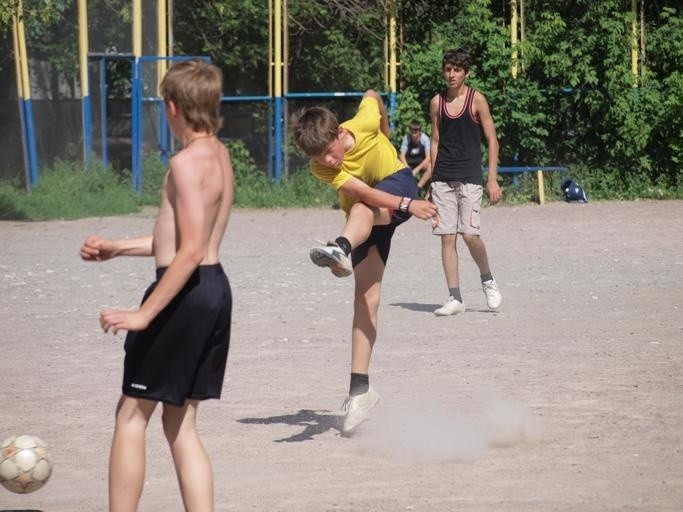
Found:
[399,197,412,212]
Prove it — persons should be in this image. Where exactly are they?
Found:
[295,90,439,434]
[399,120,432,200]
[430,48,501,317]
[79,58,236,512]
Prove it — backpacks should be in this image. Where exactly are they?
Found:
[561,177,587,202]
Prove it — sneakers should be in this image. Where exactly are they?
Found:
[310,241,352,278]
[482,276,501,309]
[434,296,465,316]
[343,388,379,433]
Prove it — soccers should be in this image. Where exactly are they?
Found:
[1,435,53,495]
